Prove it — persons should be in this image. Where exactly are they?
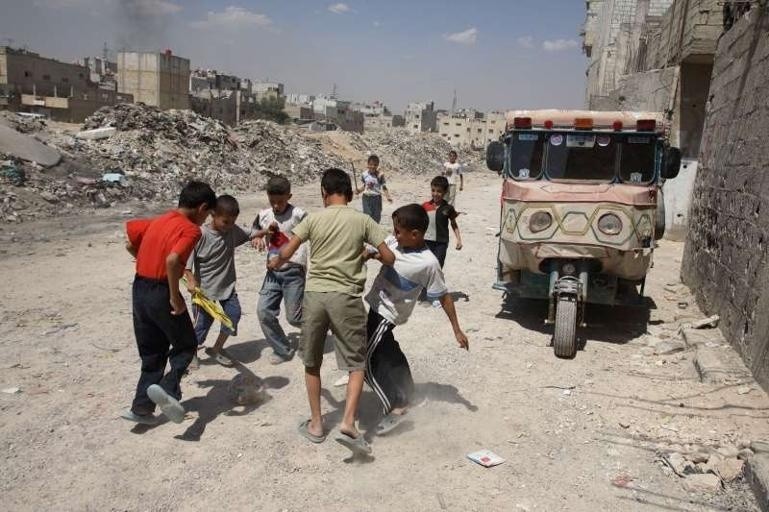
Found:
[266,168,396,454]
[361,202,470,437]
[251,176,309,365]
[184,195,277,367]
[118,181,216,426]
[354,155,392,225]
[417,176,463,309]
[441,151,464,206]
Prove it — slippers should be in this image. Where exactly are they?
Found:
[335,434,375,463]
[205,346,239,368]
[268,353,286,365]
[122,411,163,428]
[297,420,326,443]
[374,408,415,435]
[147,384,186,424]
[186,350,198,370]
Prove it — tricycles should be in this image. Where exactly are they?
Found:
[487,110,682,359]
